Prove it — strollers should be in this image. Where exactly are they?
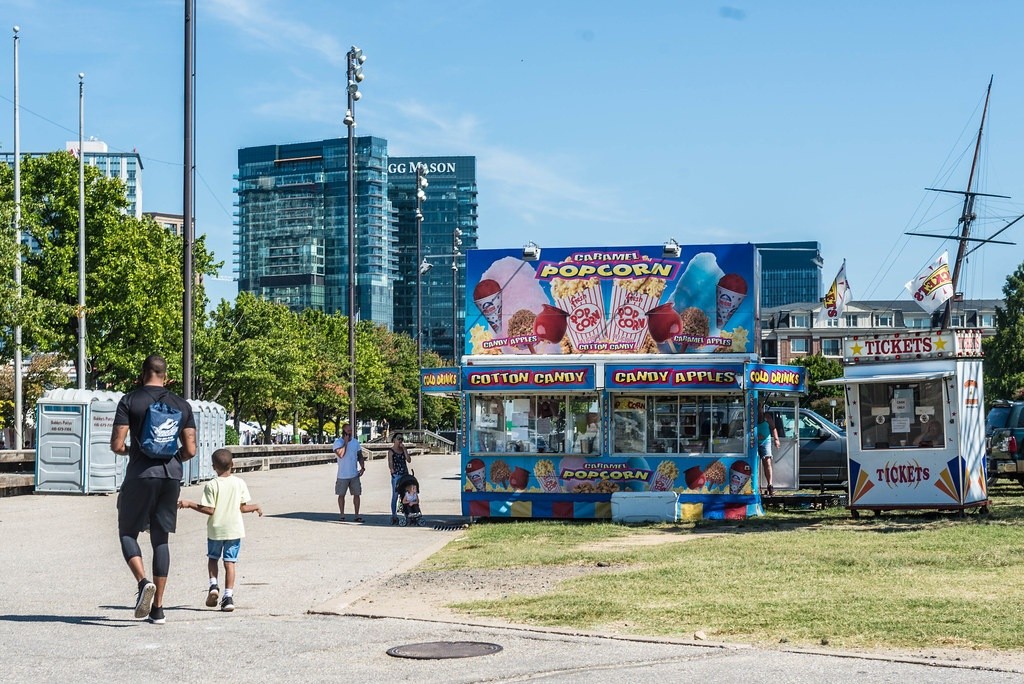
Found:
[391,469,426,527]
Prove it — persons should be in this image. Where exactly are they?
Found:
[529,402,543,436]
[713,412,729,438]
[0,441,8,450]
[388,433,411,525]
[478,397,503,452]
[912,421,944,445]
[402,483,420,519]
[272,439,277,444]
[110,355,196,624]
[252,439,260,445]
[22,441,33,449]
[656,425,685,453]
[177,449,263,612]
[333,424,364,522]
[303,437,314,444]
[758,406,781,493]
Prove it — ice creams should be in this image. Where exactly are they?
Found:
[729,460,751,494]
[715,274,747,328]
[474,278,503,334]
[465,459,486,492]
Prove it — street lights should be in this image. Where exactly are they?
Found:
[342,44,367,438]
[414,162,429,440]
[451,227,463,430]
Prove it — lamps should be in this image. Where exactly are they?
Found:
[522,239,542,262]
[420,254,467,275]
[661,237,682,258]
[757,247,824,269]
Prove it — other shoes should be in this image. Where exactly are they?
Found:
[206,584,219,607]
[392,515,398,522]
[416,514,421,517]
[408,514,413,518]
[219,597,234,612]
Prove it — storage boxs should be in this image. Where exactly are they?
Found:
[992,450,1011,459]
[610,491,679,524]
[998,462,1016,472]
[580,439,594,454]
[1016,460,1024,473]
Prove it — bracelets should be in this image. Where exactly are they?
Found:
[361,468,365,472]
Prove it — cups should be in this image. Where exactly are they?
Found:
[900,440,906,446]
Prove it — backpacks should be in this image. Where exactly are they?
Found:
[130,388,182,459]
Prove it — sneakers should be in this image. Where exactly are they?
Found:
[134,578,156,618]
[148,603,166,624]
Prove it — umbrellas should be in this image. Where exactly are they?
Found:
[227,419,260,435]
[285,424,308,436]
[249,422,278,435]
[274,425,293,436]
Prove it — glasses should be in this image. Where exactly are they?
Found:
[395,437,404,441]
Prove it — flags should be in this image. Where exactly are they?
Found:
[904,251,953,315]
[814,264,854,326]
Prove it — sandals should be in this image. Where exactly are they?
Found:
[354,517,365,522]
[339,517,346,521]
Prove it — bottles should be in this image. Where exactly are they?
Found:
[559,443,563,451]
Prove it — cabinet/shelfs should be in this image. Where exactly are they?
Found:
[654,396,700,440]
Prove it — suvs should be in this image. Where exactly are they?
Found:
[985,400,1024,487]
[647,404,848,489]
